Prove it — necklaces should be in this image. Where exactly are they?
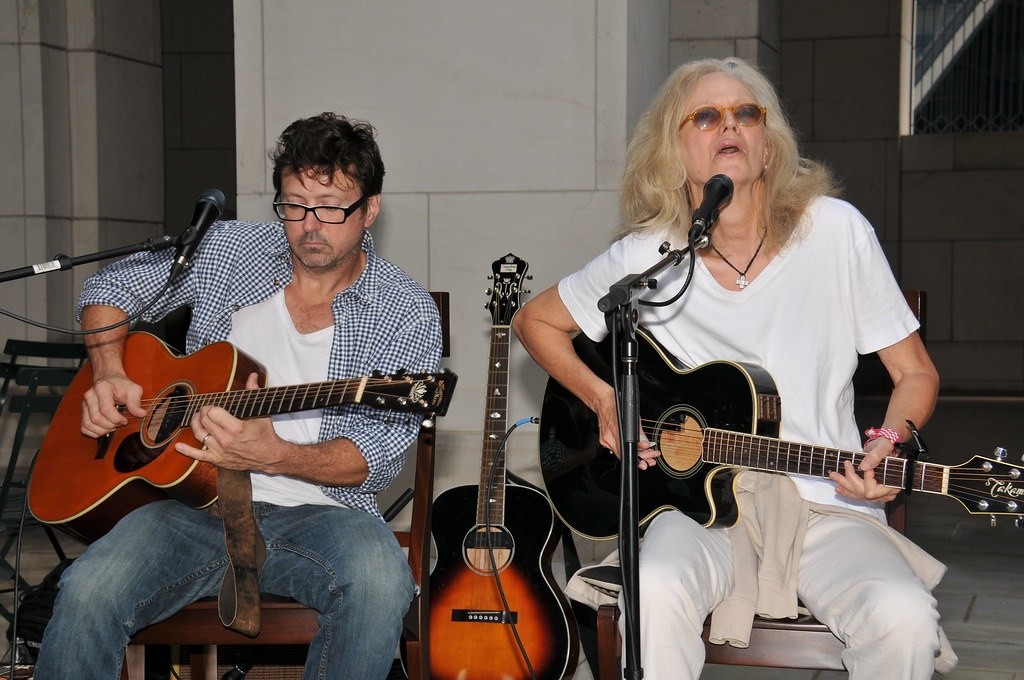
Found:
[691,207,768,289]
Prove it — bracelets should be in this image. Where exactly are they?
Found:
[863,427,902,458]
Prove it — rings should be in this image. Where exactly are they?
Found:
[202,434,211,445]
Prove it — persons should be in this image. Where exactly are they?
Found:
[510,57,941,680]
[30,111,444,680]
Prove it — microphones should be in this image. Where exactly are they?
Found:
[688,174,734,242]
[167,188,226,281]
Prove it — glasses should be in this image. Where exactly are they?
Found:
[271,189,372,224]
[676,102,768,132]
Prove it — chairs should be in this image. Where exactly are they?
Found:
[121,291,451,680]
[0,338,89,623]
[596,290,931,680]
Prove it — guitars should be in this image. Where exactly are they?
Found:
[395,253,582,680]
[538,323,1024,543]
[25,329,460,547]
